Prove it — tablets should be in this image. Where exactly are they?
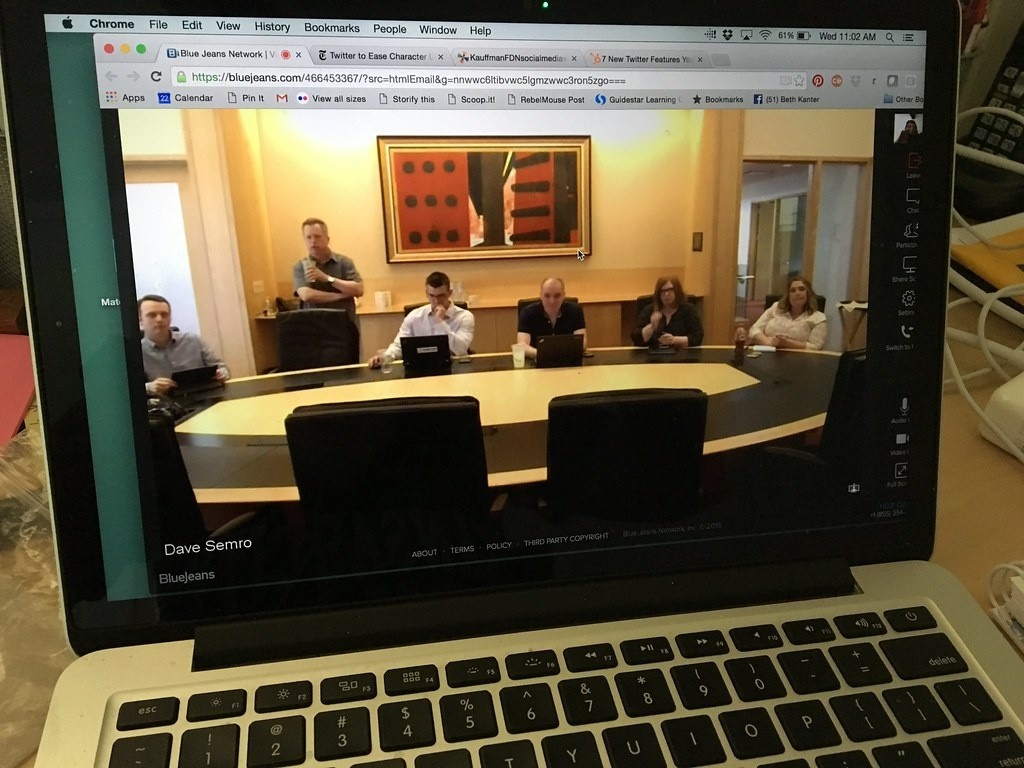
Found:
[170,363,218,396]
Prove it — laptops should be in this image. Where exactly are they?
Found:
[399,335,454,371]
[0,0,1024,768]
[533,334,584,369]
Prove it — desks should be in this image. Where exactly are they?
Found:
[171,344,847,510]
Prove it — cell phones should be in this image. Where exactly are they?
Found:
[659,344,669,348]
[747,352,761,357]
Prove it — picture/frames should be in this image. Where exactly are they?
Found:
[376,133,592,265]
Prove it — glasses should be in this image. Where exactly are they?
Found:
[657,288,675,296]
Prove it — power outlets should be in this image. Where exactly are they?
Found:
[692,232,703,251]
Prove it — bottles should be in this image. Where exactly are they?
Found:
[733,323,747,354]
[449,282,465,301]
[265,297,271,318]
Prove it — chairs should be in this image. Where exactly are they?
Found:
[285,394,490,540]
[764,347,867,496]
[258,304,360,376]
[404,302,474,356]
[517,297,577,327]
[146,414,296,561]
[636,294,695,321]
[764,293,826,314]
[547,388,708,527]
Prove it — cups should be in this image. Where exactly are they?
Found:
[375,292,391,307]
[301,261,317,282]
[510,344,526,368]
[377,348,393,374]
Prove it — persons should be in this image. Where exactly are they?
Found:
[748,276,828,349]
[368,271,475,368]
[293,218,364,365]
[630,275,705,347]
[517,277,588,359]
[137,295,231,395]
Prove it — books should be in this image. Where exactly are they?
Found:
[0,334,36,449]
[952,212,1024,307]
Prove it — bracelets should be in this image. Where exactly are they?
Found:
[327,275,335,285]
